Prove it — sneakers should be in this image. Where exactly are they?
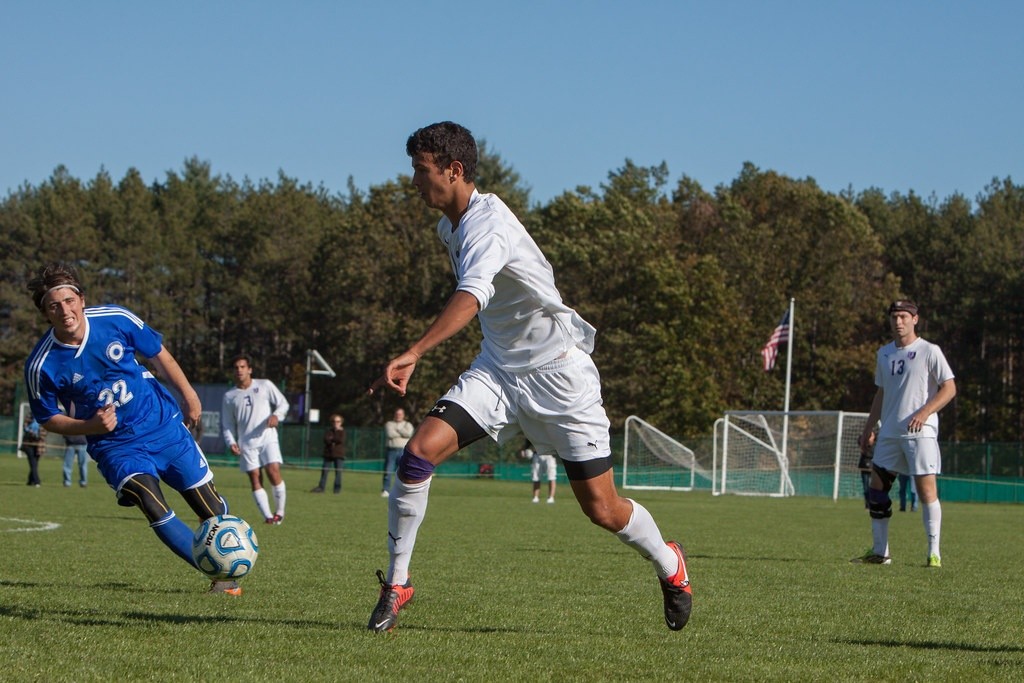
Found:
[928,554,942,567]
[367,569,414,633]
[211,579,240,596]
[658,541,692,631]
[849,551,891,566]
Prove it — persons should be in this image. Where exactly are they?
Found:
[382,407,415,497]
[857,422,881,514]
[19,412,47,488]
[366,122,693,631]
[897,474,918,512]
[309,414,347,493]
[850,299,957,567]
[24,262,242,597]
[62,435,88,486]
[221,354,290,526]
[523,439,558,503]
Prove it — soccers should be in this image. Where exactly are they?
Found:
[192,514,259,581]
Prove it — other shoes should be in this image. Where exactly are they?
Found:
[264,515,283,525]
[547,498,553,503]
[532,497,538,504]
[381,492,388,497]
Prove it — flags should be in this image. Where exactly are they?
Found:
[761,309,789,371]
